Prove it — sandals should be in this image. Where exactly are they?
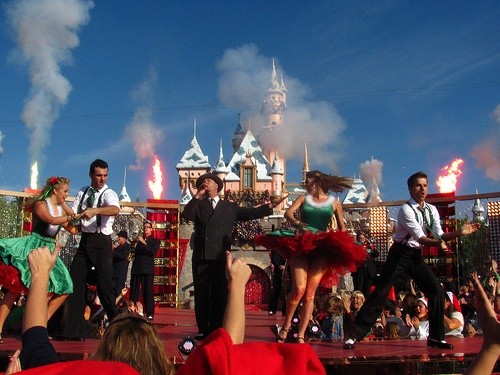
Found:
[294,337,304,344]
[276,327,290,343]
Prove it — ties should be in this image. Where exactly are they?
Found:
[417,206,429,234]
[211,198,216,210]
[87,188,99,208]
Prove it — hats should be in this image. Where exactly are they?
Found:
[447,291,461,312]
[196,173,223,193]
[116,230,129,239]
[417,297,428,308]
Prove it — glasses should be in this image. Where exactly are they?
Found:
[143,226,152,229]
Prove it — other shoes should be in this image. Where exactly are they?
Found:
[0,333,4,344]
[48,336,52,339]
[195,334,204,341]
[67,336,85,341]
[427,337,452,350]
[148,312,154,319]
[269,311,273,315]
[344,335,357,349]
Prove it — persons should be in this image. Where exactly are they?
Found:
[344,172,481,351]
[253,170,369,343]
[52,157,120,341]
[181,173,289,339]
[0,175,85,351]
[0,159,500,375]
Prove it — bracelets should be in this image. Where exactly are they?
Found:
[271,199,277,208]
[66,215,68,222]
[71,215,72,221]
[291,219,302,229]
[73,214,75,220]
